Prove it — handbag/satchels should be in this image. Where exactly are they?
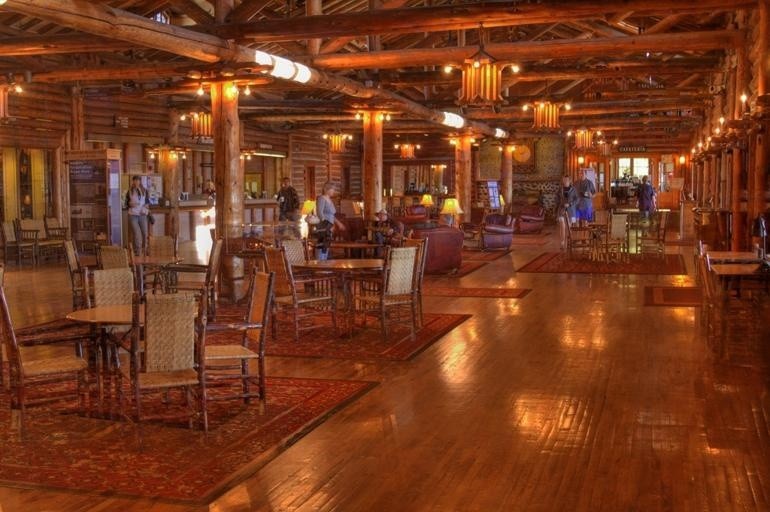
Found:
[150,216,155,224]
[307,220,333,254]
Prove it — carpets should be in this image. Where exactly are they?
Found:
[1,371,380,506]
[644,285,704,307]
[516,252,687,275]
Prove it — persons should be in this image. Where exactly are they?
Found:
[572,169,596,229]
[315,180,346,260]
[552,174,581,250]
[635,175,658,217]
[146,177,159,205]
[125,175,151,256]
[199,179,216,242]
[274,176,300,248]
[355,209,400,259]
[622,172,629,184]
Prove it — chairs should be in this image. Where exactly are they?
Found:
[558,202,671,262]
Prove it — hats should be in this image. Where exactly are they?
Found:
[374,209,389,216]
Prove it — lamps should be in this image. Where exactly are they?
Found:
[421,194,434,219]
